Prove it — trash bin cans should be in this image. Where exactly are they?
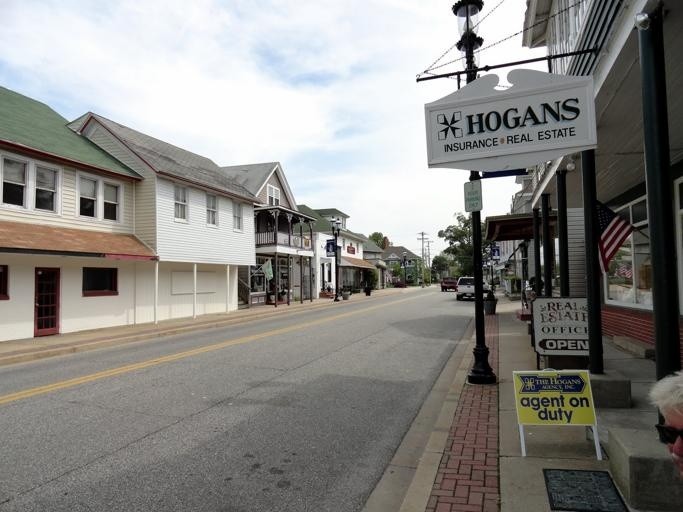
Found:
[365,287,370,296]
[266,292,276,305]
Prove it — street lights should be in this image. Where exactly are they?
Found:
[453,0,497,385]
[402,250,407,288]
[329,215,341,302]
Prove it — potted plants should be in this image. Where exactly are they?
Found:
[516,275,522,292]
[342,287,351,300]
[483,290,498,315]
[502,275,515,294]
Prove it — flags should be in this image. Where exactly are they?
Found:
[261,258,273,281]
[596,198,636,275]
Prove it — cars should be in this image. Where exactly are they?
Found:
[456,277,476,301]
[483,279,496,292]
[441,277,458,292]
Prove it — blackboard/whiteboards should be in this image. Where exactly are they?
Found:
[531,297,590,356]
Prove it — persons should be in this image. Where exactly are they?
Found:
[648,371,683,481]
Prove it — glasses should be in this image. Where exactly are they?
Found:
[655,423,683,444]
